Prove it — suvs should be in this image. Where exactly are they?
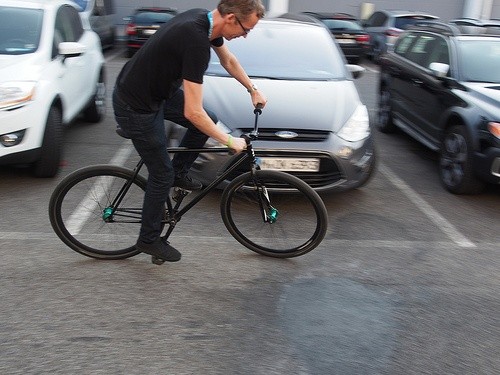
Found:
[72,0,118,48]
[0,0,110,179]
[375,18,500,196]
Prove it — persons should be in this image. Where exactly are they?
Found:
[112,0,264,262]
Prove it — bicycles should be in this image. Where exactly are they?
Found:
[47,103,329,266]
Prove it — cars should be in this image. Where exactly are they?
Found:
[299,12,372,64]
[121,6,179,57]
[167,12,378,198]
[360,8,442,64]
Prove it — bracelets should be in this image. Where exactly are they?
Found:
[247,84,256,92]
[224,134,232,149]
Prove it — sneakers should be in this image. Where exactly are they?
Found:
[134,236,182,262]
[170,174,202,191]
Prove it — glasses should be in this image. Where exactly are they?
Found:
[228,11,251,36]
